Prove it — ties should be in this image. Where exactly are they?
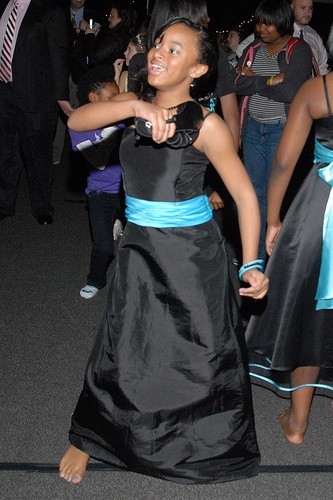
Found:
[0,1,19,83]
[71,11,78,29]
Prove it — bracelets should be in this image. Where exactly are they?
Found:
[238,259,264,280]
[270,75,274,86]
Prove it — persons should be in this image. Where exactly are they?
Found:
[65,0,158,103]
[224,1,329,76]
[127,1,241,235]
[0,0,75,225]
[56,17,270,483]
[244,25,333,445]
[67,72,141,299]
[232,2,313,276]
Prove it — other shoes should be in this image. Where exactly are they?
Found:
[0,213,16,220]
[37,214,53,225]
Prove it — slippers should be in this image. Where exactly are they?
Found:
[135,101,204,147]
